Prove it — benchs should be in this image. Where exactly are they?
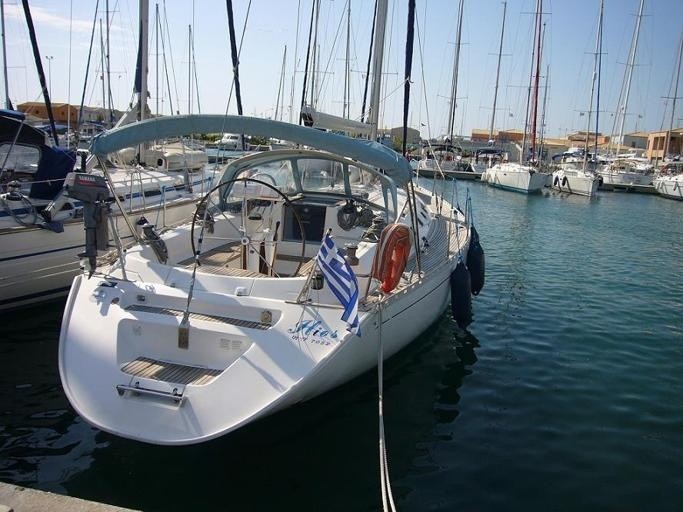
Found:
[178,240,272,277]
[293,257,316,277]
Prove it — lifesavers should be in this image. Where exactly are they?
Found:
[372,225,412,293]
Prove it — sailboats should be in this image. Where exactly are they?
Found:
[0,0,682,201]
[0,0,234,323]
[57,1,484,447]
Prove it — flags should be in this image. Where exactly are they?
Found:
[316,232,362,338]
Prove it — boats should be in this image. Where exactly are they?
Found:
[478,186,682,222]
